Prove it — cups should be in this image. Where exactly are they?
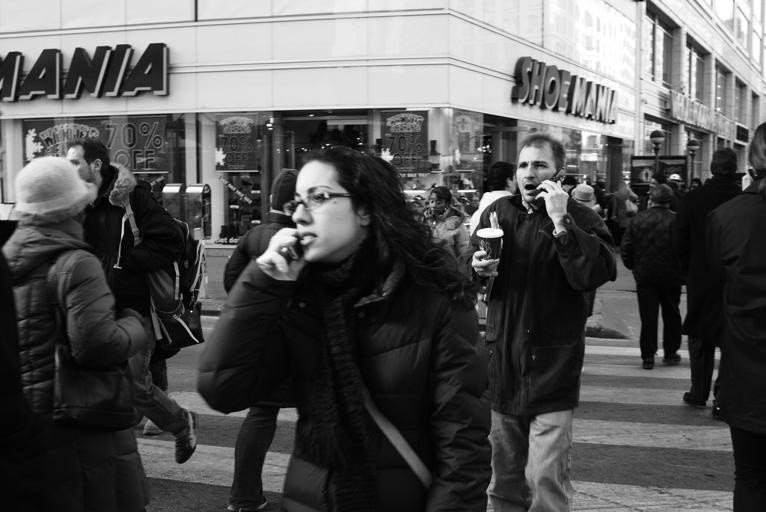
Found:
[475,227,503,260]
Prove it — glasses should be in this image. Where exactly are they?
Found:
[281,191,352,217]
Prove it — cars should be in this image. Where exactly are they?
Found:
[557,171,631,193]
[402,173,475,203]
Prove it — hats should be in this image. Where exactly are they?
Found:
[10,155,98,225]
[571,185,597,206]
[271,168,298,211]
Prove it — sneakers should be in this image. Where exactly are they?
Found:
[175,411,196,464]
[643,358,653,369]
[683,392,706,406]
[662,353,680,363]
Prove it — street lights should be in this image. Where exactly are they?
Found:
[649,128,665,178]
[685,137,702,186]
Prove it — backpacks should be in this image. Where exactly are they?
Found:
[112,201,206,310]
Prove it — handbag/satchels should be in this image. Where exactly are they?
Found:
[158,306,203,350]
[51,250,144,442]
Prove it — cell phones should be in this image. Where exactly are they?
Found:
[280,236,304,265]
[532,168,566,206]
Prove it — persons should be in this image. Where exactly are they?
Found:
[669,148,743,421]
[620,186,682,369]
[68,139,195,464]
[223,170,300,512]
[461,135,617,511]
[196,147,493,511]
[689,124,765,512]
[1,156,155,512]
[559,173,704,218]
[1,252,50,512]
[410,161,516,260]
